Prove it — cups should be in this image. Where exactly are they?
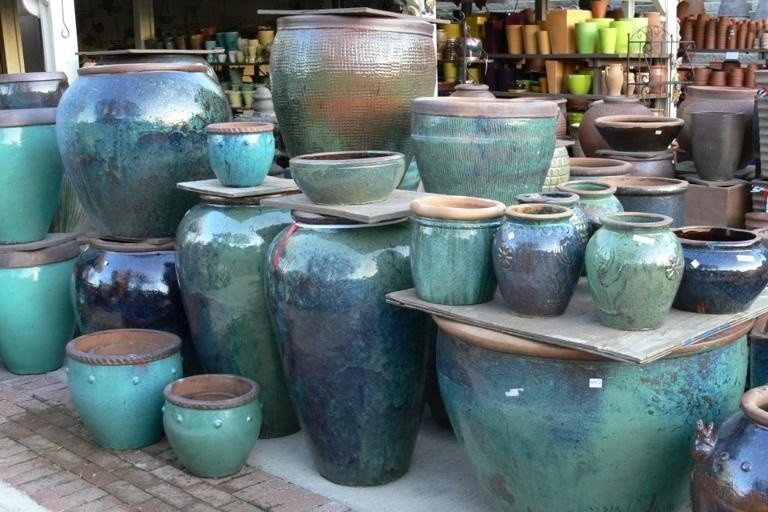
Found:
[434,0,768,132]
[143,24,276,112]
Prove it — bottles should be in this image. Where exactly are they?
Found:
[688,384,768,512]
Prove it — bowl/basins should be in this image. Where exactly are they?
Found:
[589,113,686,152]
[287,146,408,208]
